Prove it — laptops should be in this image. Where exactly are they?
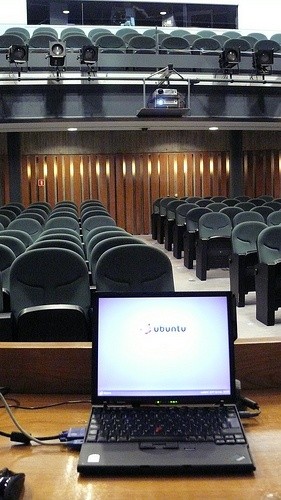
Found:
[77,291,255,476]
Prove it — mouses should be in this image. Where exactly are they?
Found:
[0,468,25,500]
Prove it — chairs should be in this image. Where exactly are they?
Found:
[150,194,281,326]
[0,27,281,57]
[1,201,174,342]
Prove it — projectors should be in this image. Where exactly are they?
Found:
[152,89,177,97]
[147,95,180,108]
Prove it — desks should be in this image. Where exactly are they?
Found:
[0,337,281,499]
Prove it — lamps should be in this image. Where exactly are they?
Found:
[77,45,99,80]
[214,45,241,83]
[250,48,275,84]
[6,44,29,81]
[45,40,66,81]
